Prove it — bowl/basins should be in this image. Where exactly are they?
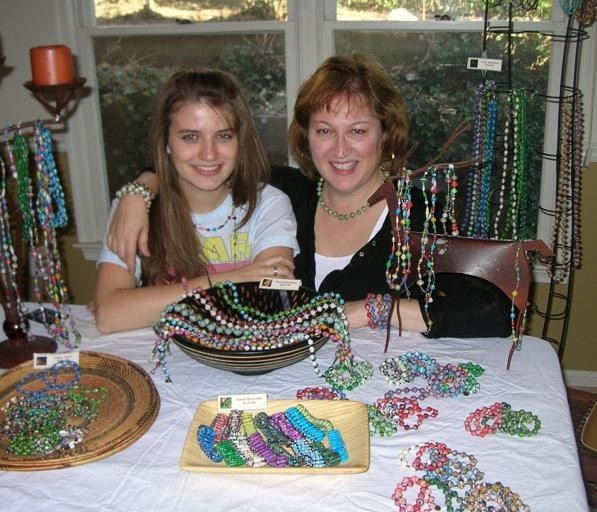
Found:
[165,280,332,375]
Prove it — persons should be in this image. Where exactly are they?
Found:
[105,50,525,341]
[92,67,302,338]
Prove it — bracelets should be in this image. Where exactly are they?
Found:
[0,359,109,457]
[363,290,396,332]
[479,0,597,27]
[295,349,542,512]
[113,180,156,213]
[180,276,189,295]
[195,403,296,468]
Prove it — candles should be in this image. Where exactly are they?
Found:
[29,42,75,85]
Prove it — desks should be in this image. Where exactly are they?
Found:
[0,300,589,511]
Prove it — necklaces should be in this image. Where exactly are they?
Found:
[460,79,586,282]
[187,205,238,235]
[0,118,83,349]
[145,278,354,379]
[384,162,459,336]
[507,238,532,352]
[313,165,392,221]
[231,212,240,272]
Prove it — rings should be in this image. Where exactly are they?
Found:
[272,266,278,276]
